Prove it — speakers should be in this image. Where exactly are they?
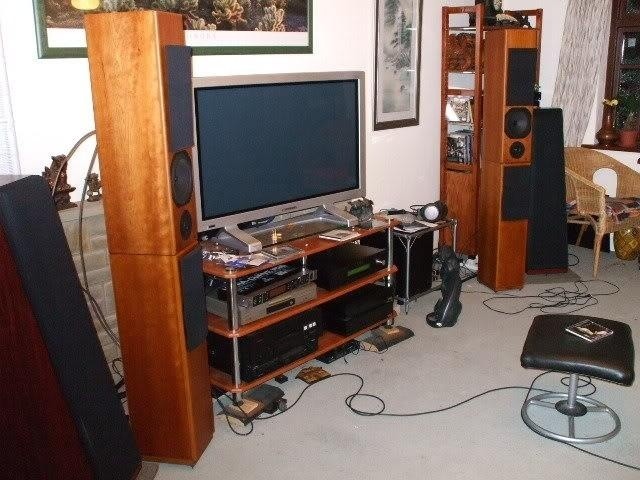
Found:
[360,221,432,299]
[105,242,215,467]
[482,29,541,164]
[528,106,571,277]
[1,176,143,480]
[80,11,215,256]
[477,159,530,293]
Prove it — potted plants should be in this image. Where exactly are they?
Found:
[619,113,639,130]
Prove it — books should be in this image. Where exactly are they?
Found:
[565,319,614,345]
[466,96,475,124]
[448,129,470,165]
[318,229,362,242]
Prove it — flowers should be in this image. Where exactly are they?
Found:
[602,97,618,107]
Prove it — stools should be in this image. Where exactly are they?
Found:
[520,314,635,443]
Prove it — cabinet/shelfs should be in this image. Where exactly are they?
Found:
[204,216,397,396]
[439,7,542,255]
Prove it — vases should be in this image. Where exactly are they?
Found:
[595,105,619,147]
[617,129,638,151]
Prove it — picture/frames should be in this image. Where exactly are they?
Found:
[373,0,423,131]
[31,0,312,58]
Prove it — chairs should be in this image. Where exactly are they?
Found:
[565,148,640,278]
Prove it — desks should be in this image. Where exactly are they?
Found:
[372,207,457,314]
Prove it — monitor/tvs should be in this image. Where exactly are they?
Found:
[191,71,365,253]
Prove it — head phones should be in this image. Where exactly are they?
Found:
[409,199,448,222]
